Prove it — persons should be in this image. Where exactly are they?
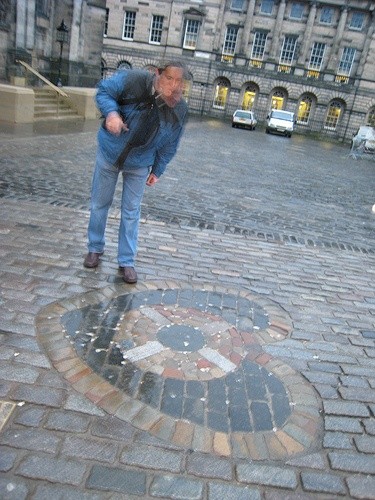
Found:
[83,59,186,284]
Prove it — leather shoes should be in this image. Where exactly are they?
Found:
[83,251,104,268]
[119,265,137,284]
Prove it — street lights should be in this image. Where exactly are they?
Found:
[55,18,70,85]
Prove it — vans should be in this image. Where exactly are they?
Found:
[266,109,297,138]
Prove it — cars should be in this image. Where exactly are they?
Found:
[232,110,257,131]
[352,125,375,154]
